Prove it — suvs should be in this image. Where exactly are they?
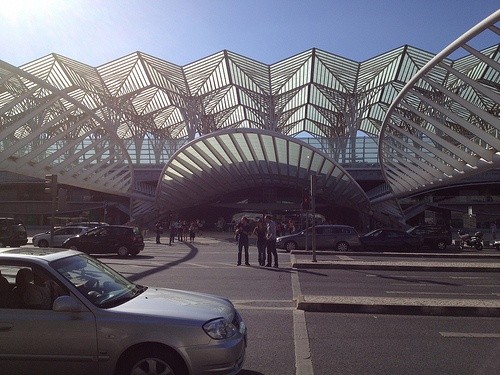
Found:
[31,225,90,250]
[408,225,453,250]
[63,226,145,258]
[0,218,29,248]
[274,224,362,253]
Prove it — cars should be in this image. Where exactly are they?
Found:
[0,247,248,375]
[358,229,422,252]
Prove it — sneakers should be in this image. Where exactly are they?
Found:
[265,263,271,267]
[272,264,278,268]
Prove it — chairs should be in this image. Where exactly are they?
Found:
[1,268,44,307]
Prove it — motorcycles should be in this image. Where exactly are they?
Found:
[455,230,499,252]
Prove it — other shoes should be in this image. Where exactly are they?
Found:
[245,263,250,266]
[258,262,265,266]
[237,262,241,266]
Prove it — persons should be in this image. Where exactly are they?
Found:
[235,217,250,265]
[155,217,338,244]
[253,219,268,266]
[264,216,278,269]
[491,223,498,240]
[75,279,96,293]
